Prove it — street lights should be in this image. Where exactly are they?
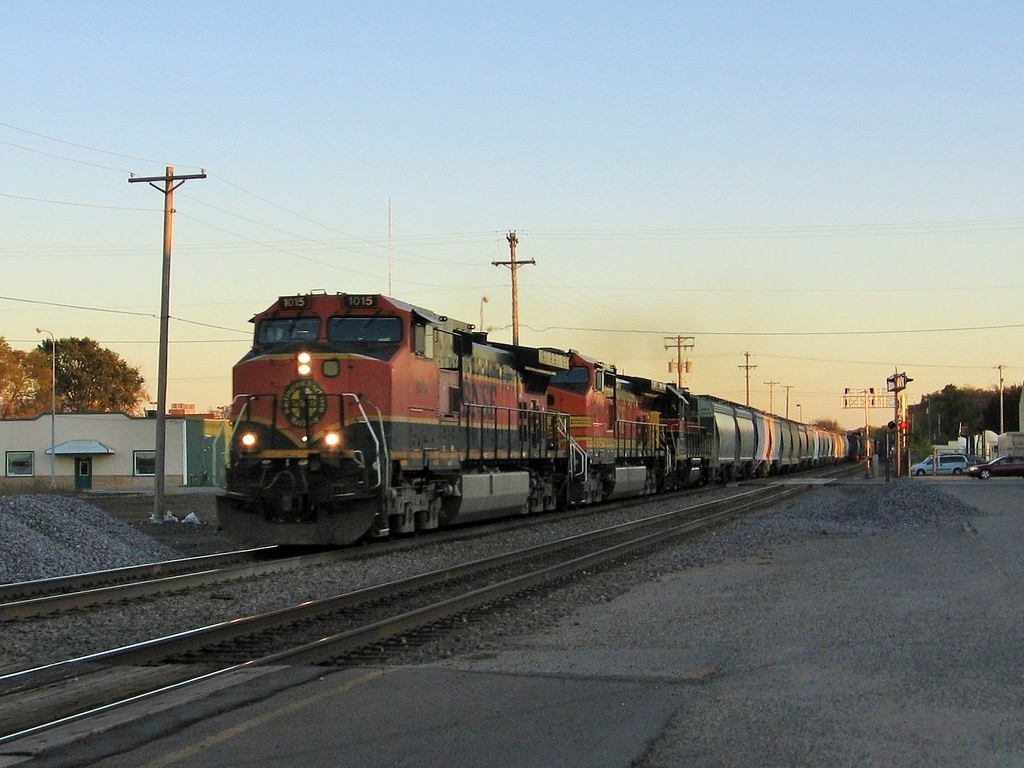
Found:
[796,403,804,422]
[34,326,59,488]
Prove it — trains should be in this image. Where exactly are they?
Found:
[211,291,853,561]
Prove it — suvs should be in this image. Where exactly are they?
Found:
[911,452,968,476]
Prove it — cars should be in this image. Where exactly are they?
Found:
[965,454,988,466]
[966,454,1024,486]
